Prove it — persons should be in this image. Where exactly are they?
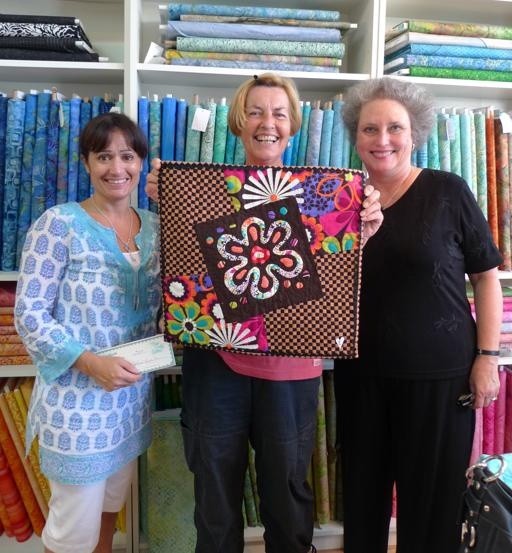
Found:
[338,77,503,553]
[143,71,387,553]
[16,112,182,553]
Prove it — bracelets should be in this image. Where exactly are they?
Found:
[476,348,501,355]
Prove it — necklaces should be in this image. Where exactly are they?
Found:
[90,194,134,252]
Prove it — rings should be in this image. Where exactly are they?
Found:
[492,397,497,401]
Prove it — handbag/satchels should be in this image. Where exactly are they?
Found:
[457,454,511,552]
